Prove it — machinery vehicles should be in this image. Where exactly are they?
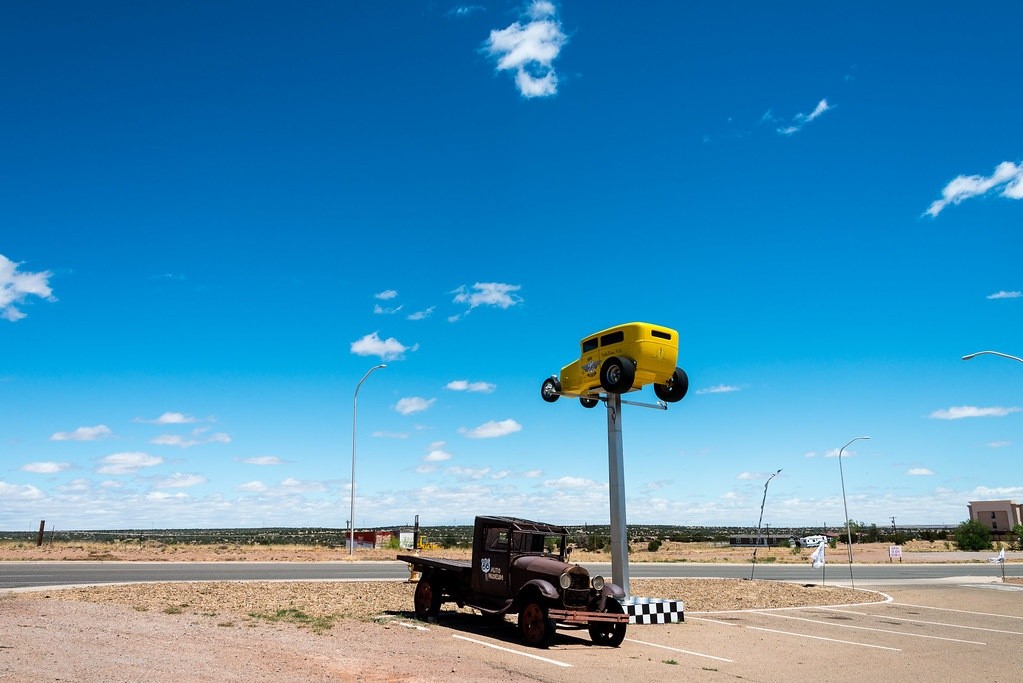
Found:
[417,536,444,550]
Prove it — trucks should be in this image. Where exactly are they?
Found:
[800,535,828,548]
[395,515,626,648]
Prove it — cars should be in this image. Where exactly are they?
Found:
[540,322,688,409]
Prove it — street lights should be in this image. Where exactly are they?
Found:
[839,437,871,589]
[349,364,388,554]
